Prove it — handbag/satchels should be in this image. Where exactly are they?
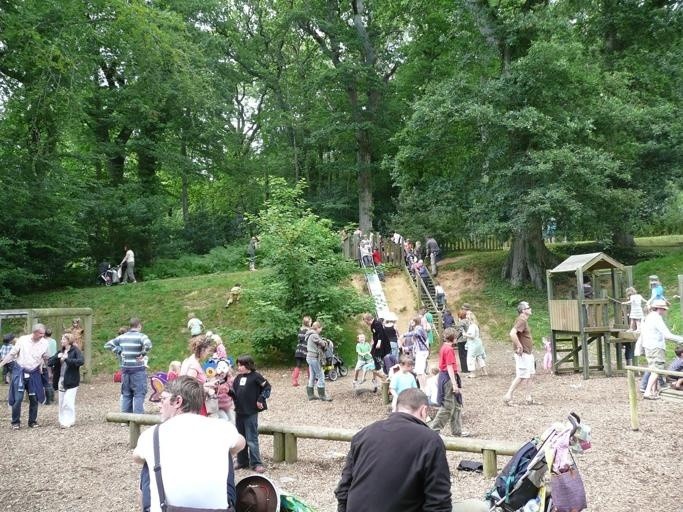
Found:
[551,463,588,512]
[113,372,121,382]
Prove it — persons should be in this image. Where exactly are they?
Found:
[581,273,683,402]
[117,245,136,285]
[0,322,84,430]
[352,227,445,305]
[501,301,537,407]
[246,235,259,271]
[332,387,453,511]
[223,282,241,307]
[540,335,554,373]
[132,375,248,511]
[102,312,272,475]
[292,304,488,436]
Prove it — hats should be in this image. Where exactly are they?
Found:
[651,299,669,310]
[462,303,470,309]
[234,476,281,512]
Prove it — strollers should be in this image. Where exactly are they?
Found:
[459,412,580,511]
[95,263,122,287]
[317,337,348,382]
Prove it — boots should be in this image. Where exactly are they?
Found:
[307,386,319,400]
[293,367,298,385]
[318,387,332,401]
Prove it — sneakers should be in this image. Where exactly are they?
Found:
[254,465,264,472]
[526,400,542,406]
[232,463,240,469]
[451,432,468,436]
[32,423,38,427]
[13,423,20,430]
[503,399,516,407]
